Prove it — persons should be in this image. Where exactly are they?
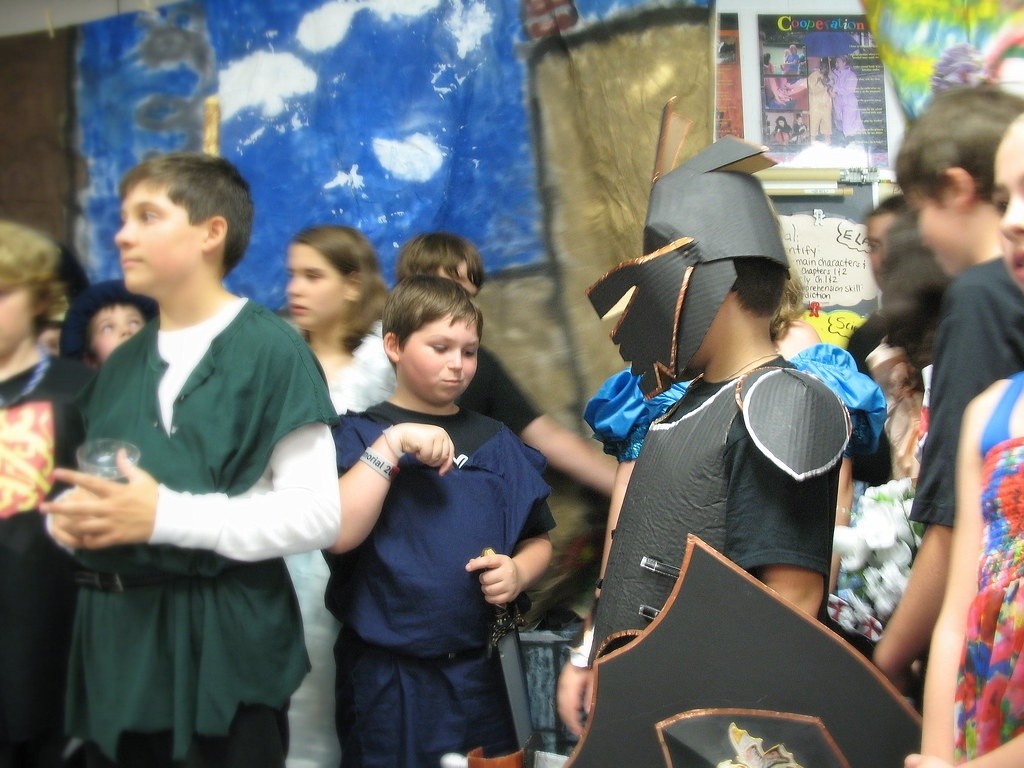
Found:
[847,81,1024,768]
[556,133,888,767]
[0,215,620,768]
[38,149,341,768]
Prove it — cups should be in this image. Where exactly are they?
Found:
[76,439,141,500]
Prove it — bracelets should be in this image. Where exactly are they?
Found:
[560,643,594,670]
[358,424,404,481]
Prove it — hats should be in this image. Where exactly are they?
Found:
[0,219,63,288]
[60,279,160,356]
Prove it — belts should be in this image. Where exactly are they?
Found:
[71,565,200,592]
